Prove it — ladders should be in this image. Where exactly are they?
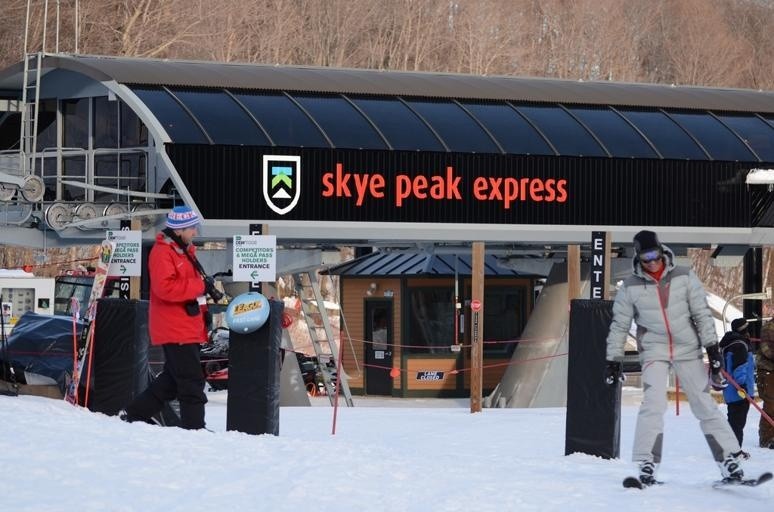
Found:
[292,271,354,407]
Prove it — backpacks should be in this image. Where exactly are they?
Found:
[708,340,748,389]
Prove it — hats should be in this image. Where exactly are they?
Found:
[633,231,664,263]
[167,205,200,229]
[732,318,749,336]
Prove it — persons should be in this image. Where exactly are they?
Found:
[114,206,212,431]
[719,317,756,458]
[603,230,759,489]
[0,305,11,324]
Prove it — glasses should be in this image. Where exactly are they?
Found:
[636,250,661,264]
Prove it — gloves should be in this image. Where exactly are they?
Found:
[602,362,624,386]
[706,348,722,373]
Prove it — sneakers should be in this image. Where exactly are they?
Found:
[640,462,656,484]
[720,451,750,479]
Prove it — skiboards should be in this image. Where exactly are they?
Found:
[65,241,117,405]
[624,473,773,489]
